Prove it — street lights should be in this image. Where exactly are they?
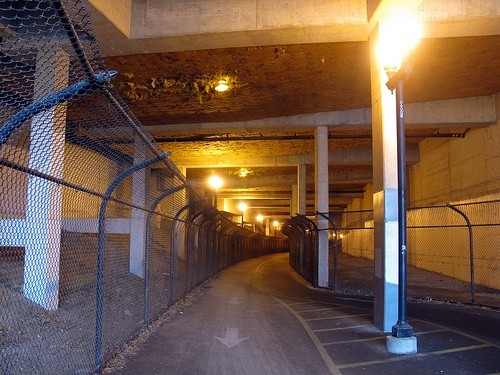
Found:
[370,4,414,338]
